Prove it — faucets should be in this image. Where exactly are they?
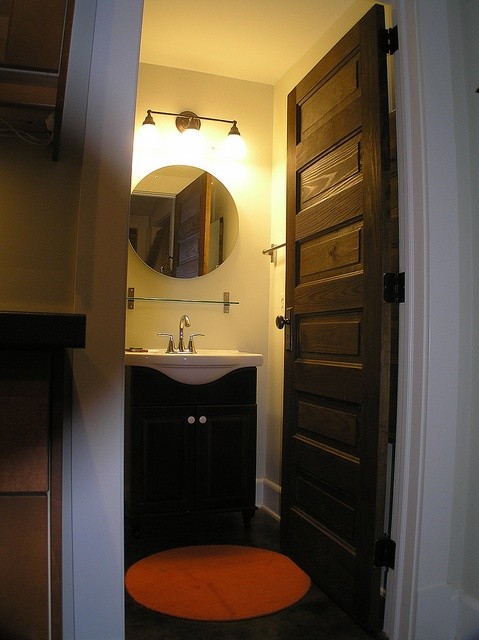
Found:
[178,313,190,352]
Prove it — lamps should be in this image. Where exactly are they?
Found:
[142,110,156,134]
[184,116,199,139]
[228,122,241,140]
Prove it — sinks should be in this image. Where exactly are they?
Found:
[125,347,264,385]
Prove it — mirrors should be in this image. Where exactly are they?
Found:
[129,165,239,279]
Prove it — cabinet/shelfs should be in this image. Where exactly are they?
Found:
[0,0,78,160]
[126,288,240,313]
[124,366,257,519]
[0,345,73,640]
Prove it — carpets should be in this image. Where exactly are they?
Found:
[125,545,312,621]
